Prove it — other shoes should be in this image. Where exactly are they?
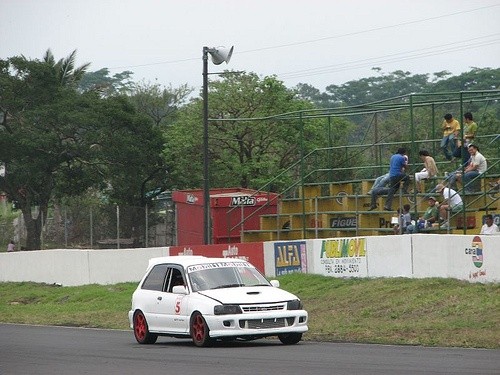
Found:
[383,206,393,210]
[434,216,447,224]
[366,205,377,210]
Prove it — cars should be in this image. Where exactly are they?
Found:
[127,256,309,347]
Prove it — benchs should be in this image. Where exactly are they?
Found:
[240,172,500,243]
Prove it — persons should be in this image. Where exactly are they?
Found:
[365,112,488,234]
[479,214,499,234]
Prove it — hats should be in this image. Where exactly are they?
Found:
[429,196,436,201]
[434,183,445,193]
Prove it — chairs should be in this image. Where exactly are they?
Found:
[166,276,180,292]
